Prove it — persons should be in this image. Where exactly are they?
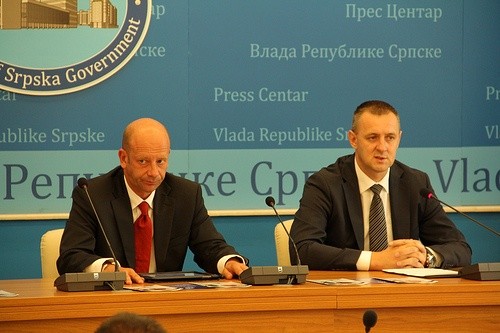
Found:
[288,99,472,271]
[56,117,249,285]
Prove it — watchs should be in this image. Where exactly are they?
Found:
[424,247,437,268]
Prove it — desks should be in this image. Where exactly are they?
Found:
[0,269,500,333]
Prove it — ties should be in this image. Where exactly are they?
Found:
[134,201,153,275]
[368,184,389,252]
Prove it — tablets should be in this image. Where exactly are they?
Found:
[139,270,220,282]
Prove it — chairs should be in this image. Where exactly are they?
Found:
[41,228,64,280]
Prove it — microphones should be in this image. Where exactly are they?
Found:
[241,196,307,285]
[54,177,126,291]
[419,190,500,280]
